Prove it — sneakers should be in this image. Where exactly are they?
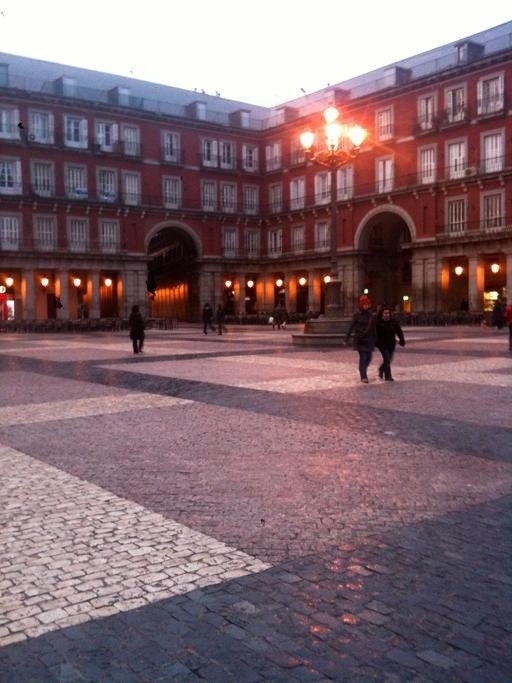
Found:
[360,368,394,383]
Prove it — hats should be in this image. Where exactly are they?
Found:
[360,294,369,308]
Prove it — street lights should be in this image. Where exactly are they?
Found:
[300,106,368,333]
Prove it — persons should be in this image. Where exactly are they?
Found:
[341,295,376,383]
[374,305,406,381]
[201,303,216,336]
[460,294,512,351]
[269,303,289,330]
[214,304,228,335]
[126,303,146,354]
